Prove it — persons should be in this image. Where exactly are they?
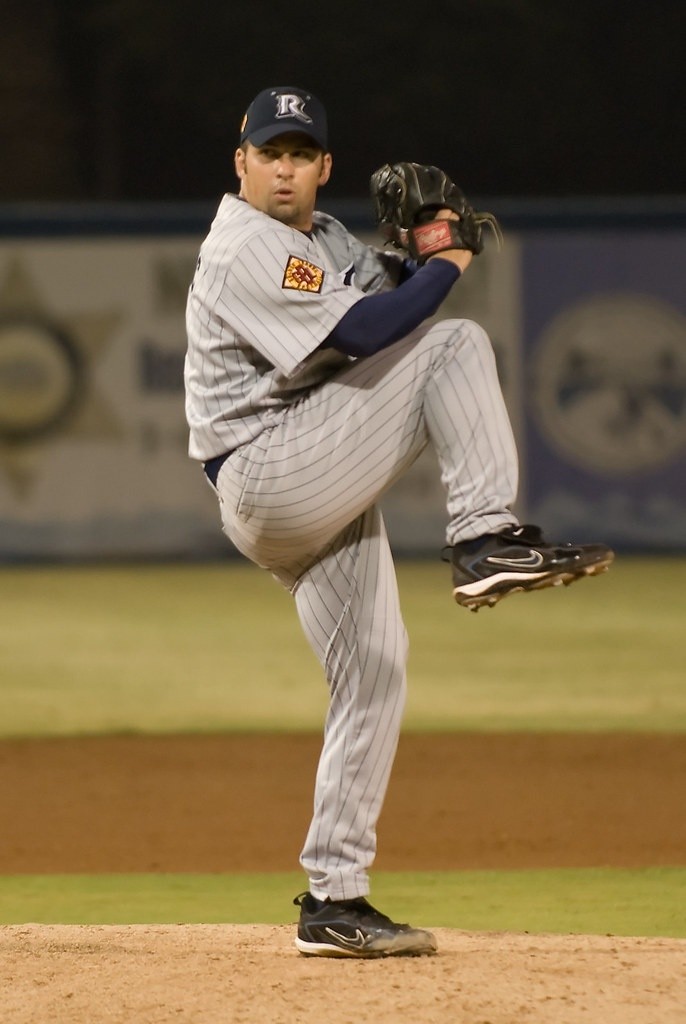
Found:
[182,86,617,959]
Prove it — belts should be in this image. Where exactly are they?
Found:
[205,449,236,489]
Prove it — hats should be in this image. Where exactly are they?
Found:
[241,87,329,152]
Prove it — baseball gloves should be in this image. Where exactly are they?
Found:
[371,161,483,268]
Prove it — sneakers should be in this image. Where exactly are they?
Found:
[293,892,439,958]
[440,524,613,616]
